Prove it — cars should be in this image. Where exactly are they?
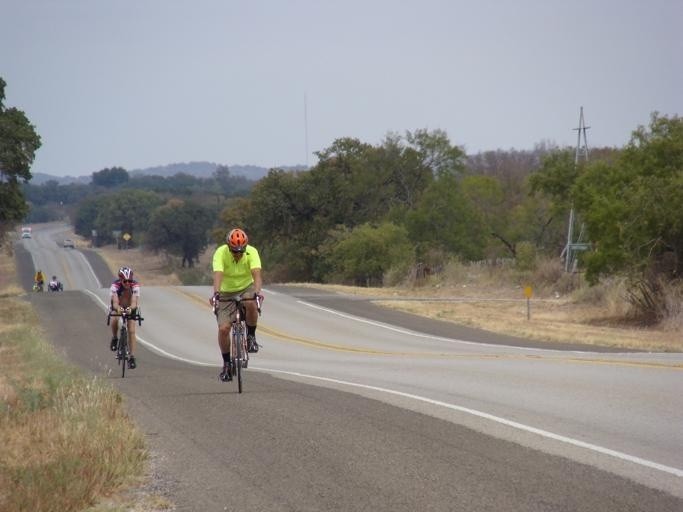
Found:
[63,239,75,248]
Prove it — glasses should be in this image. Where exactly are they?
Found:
[121,279,133,284]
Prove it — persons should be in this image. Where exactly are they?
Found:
[209,228,265,381]
[109,267,141,369]
[35,269,46,291]
[49,275,59,291]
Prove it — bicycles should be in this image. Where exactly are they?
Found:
[210,292,265,395]
[103,310,143,381]
[35,281,43,292]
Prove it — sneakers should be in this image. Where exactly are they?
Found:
[126,352,136,369]
[220,361,233,382]
[110,335,118,351]
[246,333,259,353]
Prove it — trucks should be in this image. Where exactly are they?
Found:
[20,226,32,240]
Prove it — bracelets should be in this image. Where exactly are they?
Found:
[214,291,220,295]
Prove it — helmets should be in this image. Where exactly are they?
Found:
[118,266,134,281]
[226,229,249,254]
